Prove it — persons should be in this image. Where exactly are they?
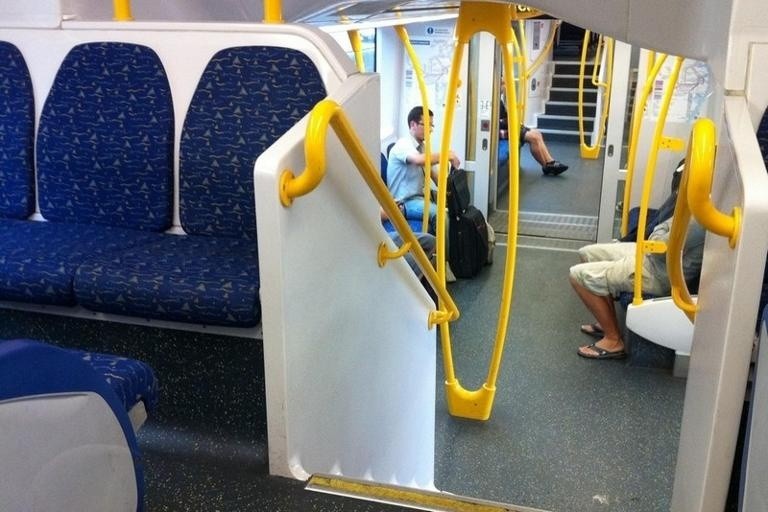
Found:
[500,75,569,177]
[386,104,460,284]
[568,213,705,360]
[382,199,437,280]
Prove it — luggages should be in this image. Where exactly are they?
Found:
[447,207,491,281]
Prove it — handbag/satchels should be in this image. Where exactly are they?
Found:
[445,164,471,217]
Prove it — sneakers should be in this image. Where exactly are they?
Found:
[541,159,568,175]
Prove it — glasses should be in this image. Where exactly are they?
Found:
[415,121,435,130]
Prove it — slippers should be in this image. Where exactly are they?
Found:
[580,321,605,337]
[576,341,628,360]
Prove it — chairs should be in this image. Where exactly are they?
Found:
[72,41,328,329]
[620,204,698,377]
[0,337,159,511]
[3,42,175,309]
[499,133,523,197]
[380,139,436,235]
[1,39,36,235]
